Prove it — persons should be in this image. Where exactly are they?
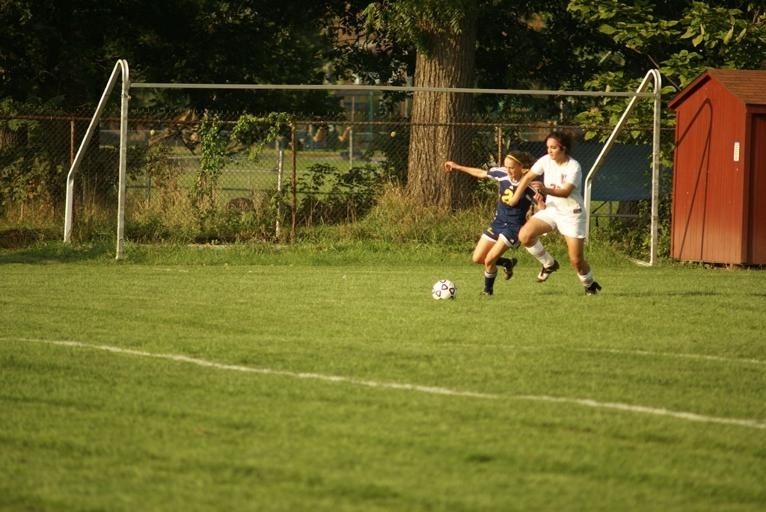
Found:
[504,132,603,296]
[442,150,547,296]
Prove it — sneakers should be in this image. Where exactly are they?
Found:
[536,259,559,283]
[503,257,517,280]
[482,291,493,295]
[584,281,602,296]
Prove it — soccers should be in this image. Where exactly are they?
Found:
[432,280,455,299]
